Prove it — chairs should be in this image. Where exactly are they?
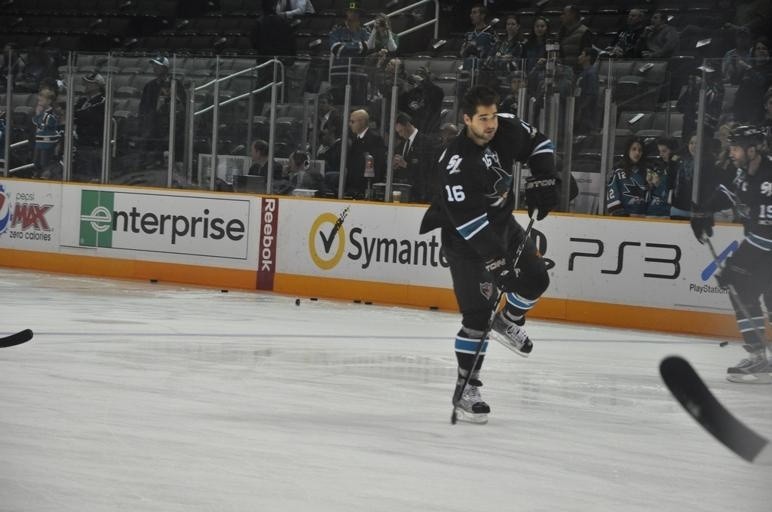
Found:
[0,0,769,217]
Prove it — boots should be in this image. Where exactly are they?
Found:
[727,343,772,373]
[453,375,490,413]
[493,308,533,353]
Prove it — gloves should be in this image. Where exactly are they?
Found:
[689,200,715,245]
[483,251,517,293]
[525,177,559,220]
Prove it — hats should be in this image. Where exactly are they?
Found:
[150,57,169,70]
[81,73,106,89]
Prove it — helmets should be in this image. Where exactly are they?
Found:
[728,126,766,145]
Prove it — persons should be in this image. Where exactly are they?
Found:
[691,124,772,373]
[419,85,559,413]
[0,0,772,223]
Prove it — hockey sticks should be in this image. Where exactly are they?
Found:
[451,207,538,423]
[660,356,772,467]
[0,329,33,347]
